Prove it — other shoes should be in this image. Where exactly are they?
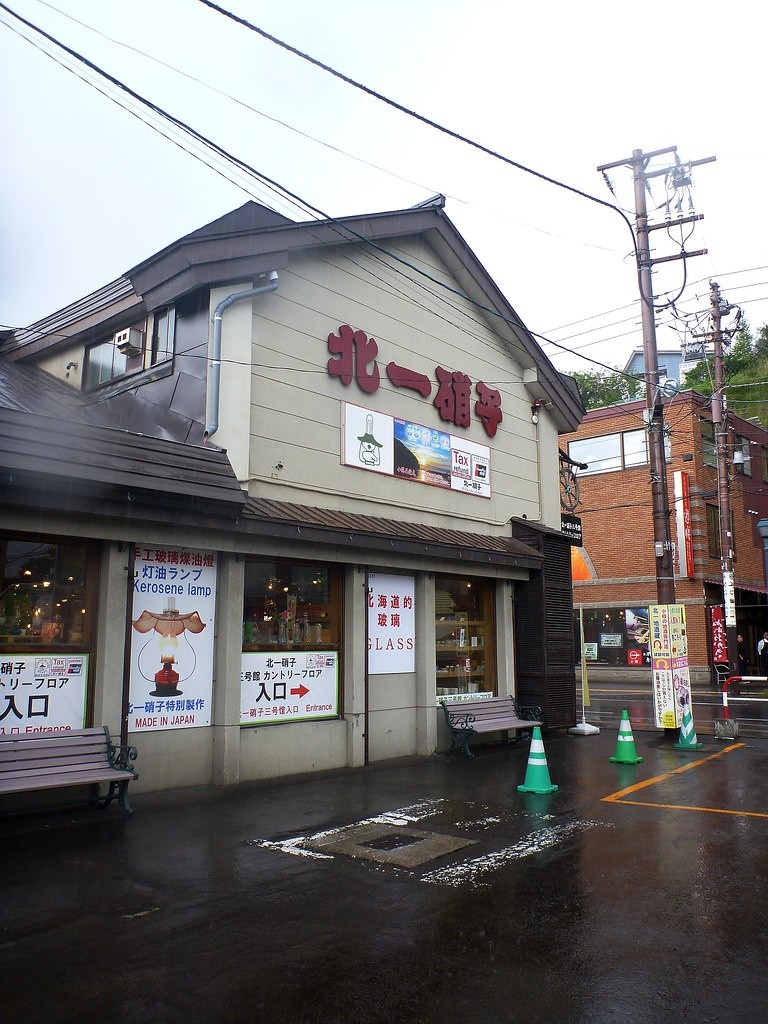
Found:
[739,680,750,684]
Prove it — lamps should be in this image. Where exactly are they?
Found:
[132,597,206,697]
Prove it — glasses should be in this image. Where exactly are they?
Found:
[764,634,768,636]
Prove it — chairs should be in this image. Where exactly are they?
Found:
[715,664,730,685]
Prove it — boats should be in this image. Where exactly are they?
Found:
[634,627,650,653]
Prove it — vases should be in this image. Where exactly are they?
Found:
[279,623,288,645]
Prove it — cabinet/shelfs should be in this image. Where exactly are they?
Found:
[435,620,485,696]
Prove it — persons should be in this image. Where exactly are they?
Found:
[757,632,768,678]
[736,635,746,675]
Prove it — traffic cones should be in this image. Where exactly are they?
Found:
[673,703,703,750]
[608,709,644,764]
[516,726,559,796]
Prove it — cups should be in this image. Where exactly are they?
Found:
[468,684,476,693]
[246,617,259,644]
[437,688,464,696]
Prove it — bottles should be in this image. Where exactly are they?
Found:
[280,617,309,643]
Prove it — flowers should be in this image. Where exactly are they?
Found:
[277,610,291,623]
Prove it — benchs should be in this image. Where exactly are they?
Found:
[440,695,547,758]
[0,726,139,817]
